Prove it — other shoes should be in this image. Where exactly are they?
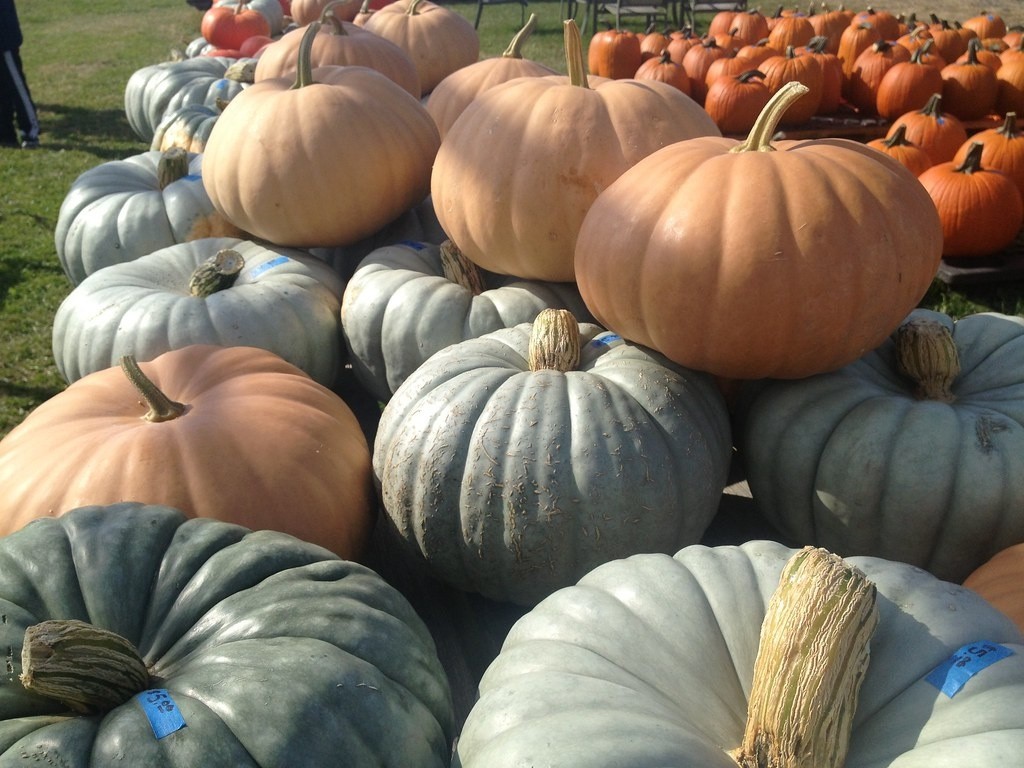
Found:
[21,138,39,148]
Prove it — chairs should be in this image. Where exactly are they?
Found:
[474,0,749,36]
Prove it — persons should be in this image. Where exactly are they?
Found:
[0,0,40,149]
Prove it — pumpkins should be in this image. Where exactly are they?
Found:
[0,0,1024,768]
